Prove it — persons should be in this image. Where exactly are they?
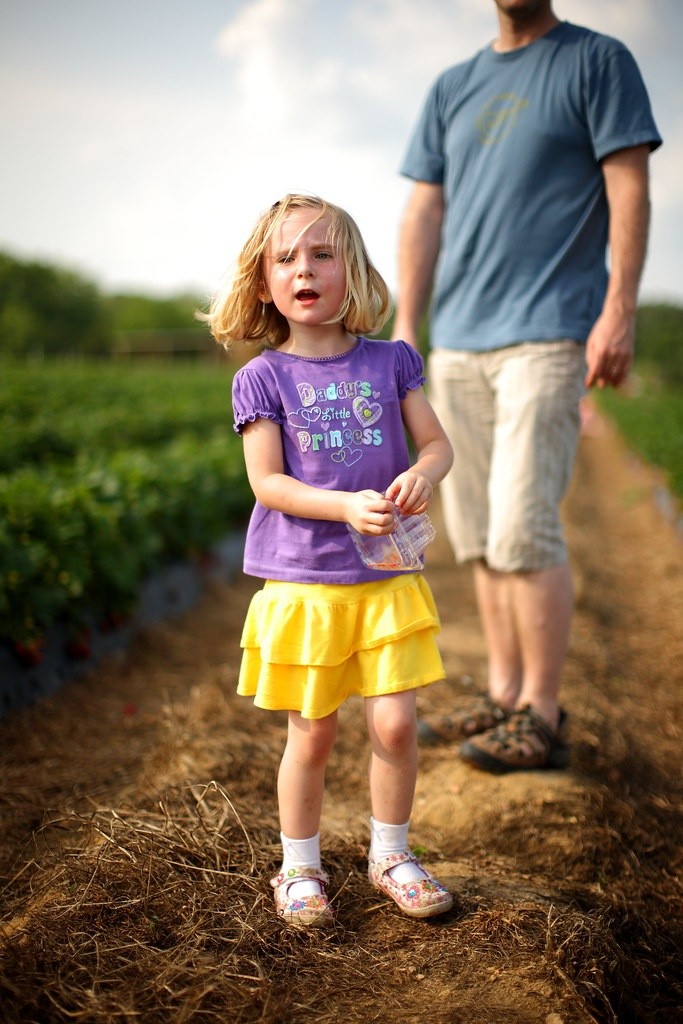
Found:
[195,194,461,928]
[387,0,661,771]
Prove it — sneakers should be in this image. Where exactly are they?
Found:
[421,687,527,744]
[464,713,576,774]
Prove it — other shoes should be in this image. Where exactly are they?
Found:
[367,854,450,920]
[269,863,335,931]
